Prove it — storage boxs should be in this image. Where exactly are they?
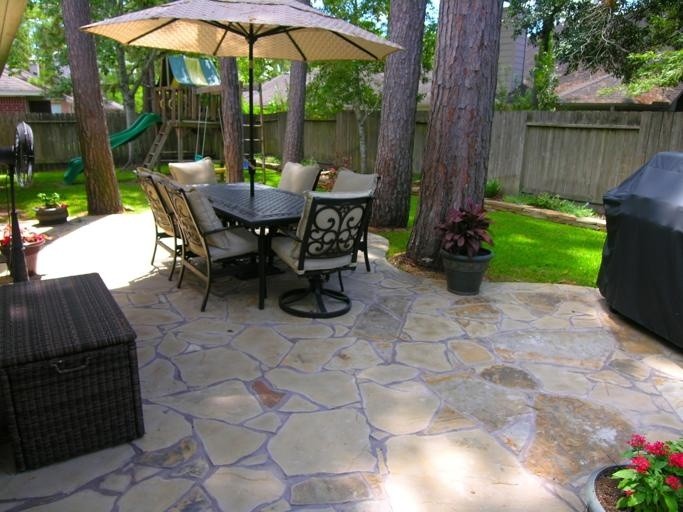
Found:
[0,272,146,475]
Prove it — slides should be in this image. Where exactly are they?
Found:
[63,113,161,185]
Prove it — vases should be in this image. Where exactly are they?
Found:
[36,209,69,225]
[583,461,630,512]
[1,239,45,278]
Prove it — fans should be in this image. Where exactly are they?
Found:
[0,120,36,284]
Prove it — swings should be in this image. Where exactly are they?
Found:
[195,93,227,174]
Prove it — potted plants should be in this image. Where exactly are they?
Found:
[431,190,496,296]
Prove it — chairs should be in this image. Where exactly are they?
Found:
[131,166,382,319]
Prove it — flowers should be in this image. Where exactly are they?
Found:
[608,432,682,512]
[36,192,71,209]
[0,222,55,252]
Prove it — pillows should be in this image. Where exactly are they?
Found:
[276,162,320,194]
[168,157,217,184]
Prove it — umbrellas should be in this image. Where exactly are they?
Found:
[77,0,408,266]
